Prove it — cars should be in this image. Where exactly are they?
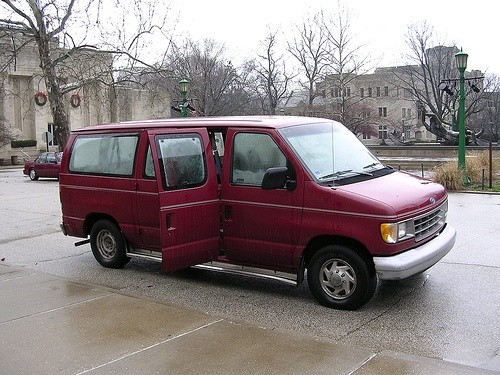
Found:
[23,152,62,181]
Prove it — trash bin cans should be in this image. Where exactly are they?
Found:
[10,156,18,164]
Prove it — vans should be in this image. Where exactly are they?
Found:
[57,116,455,310]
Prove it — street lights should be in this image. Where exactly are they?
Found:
[455,46,470,186]
[179,76,190,116]
